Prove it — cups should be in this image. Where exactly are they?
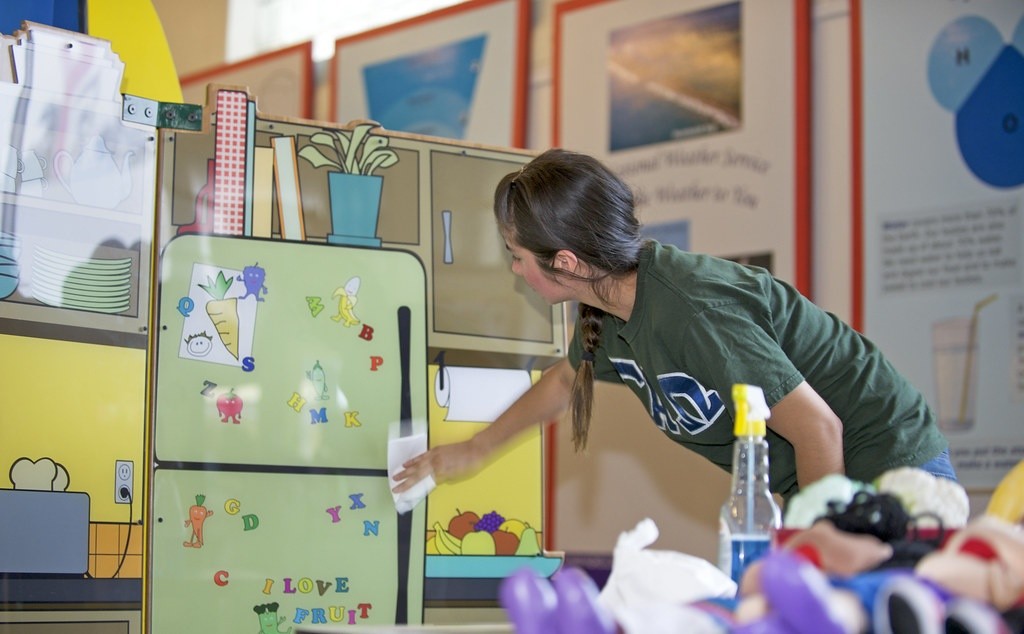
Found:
[930,318,981,434]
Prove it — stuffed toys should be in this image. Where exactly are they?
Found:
[497,495,1024,633]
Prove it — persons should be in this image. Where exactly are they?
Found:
[389,149,957,518]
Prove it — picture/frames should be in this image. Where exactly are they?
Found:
[176,38,313,130]
[846,1,1023,523]
[555,0,814,297]
[326,0,530,148]
